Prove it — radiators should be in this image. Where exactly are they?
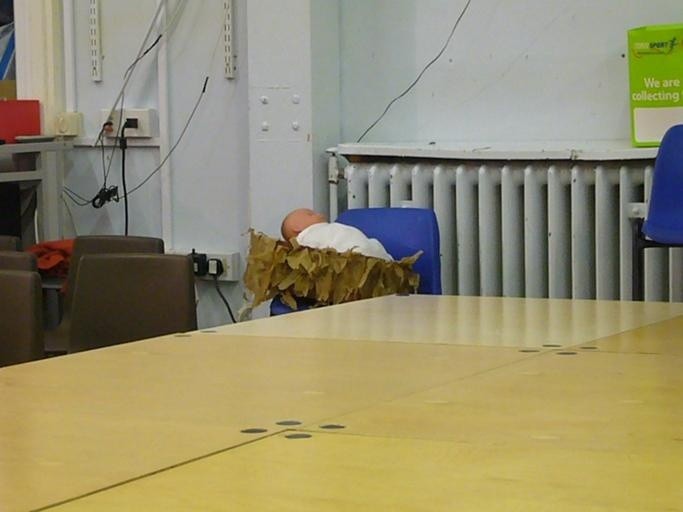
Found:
[341,159,682,305]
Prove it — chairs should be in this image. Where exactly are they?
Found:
[270,208,442,317]
[2,231,197,366]
[630,123,683,303]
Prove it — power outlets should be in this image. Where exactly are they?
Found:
[99,108,158,140]
[192,250,241,283]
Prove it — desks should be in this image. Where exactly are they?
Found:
[0,294,683,509]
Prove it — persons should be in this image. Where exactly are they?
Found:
[280,208,394,264]
